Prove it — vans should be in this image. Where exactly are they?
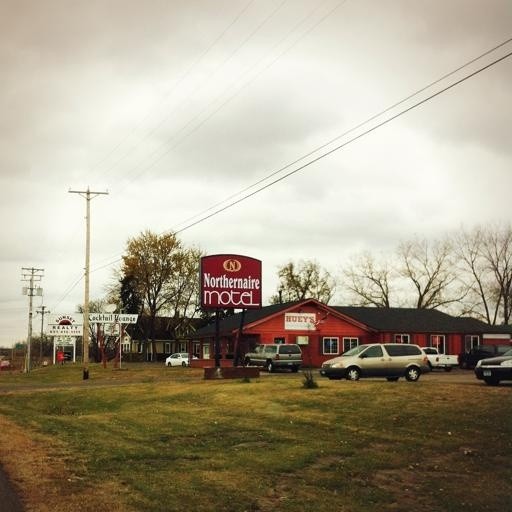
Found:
[319,342,430,382]
[243,343,304,374]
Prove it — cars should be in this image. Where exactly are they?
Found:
[165,352,198,367]
[474,348,512,386]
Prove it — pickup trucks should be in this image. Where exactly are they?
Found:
[421,346,460,372]
